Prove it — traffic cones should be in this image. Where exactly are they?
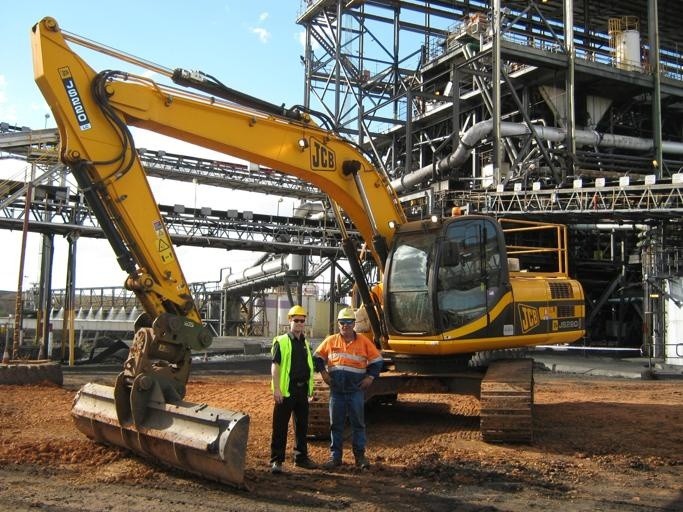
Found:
[1,346,11,364]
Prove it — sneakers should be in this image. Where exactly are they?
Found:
[271,461,281,473]
[294,458,318,468]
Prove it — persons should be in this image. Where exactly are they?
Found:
[271,304,318,474]
[312,308,384,470]
[414,96,421,117]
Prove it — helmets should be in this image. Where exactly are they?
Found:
[338,308,355,319]
[288,305,306,317]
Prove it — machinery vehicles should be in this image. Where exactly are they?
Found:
[28,14,593,494]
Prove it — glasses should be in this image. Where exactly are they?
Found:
[293,319,305,323]
[340,320,352,324]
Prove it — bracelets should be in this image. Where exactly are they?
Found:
[367,375,375,380]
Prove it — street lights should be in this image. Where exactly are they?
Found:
[37,345,45,361]
